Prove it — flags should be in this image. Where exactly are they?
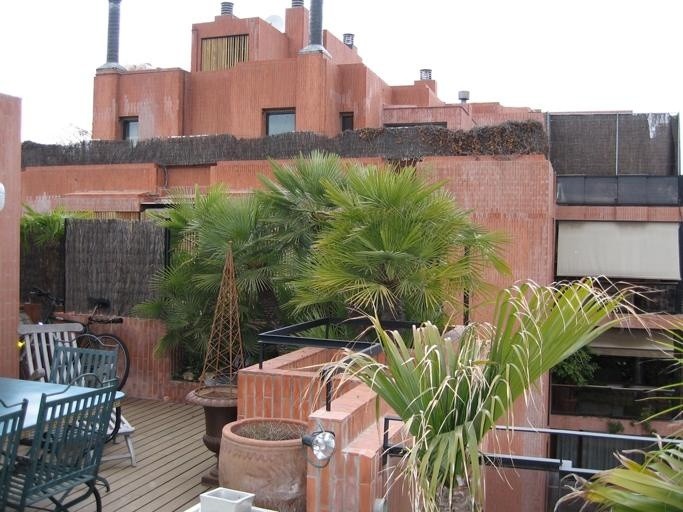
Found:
[184,385,239,487]
[198,483,257,512]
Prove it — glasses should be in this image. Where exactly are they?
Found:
[300,421,337,470]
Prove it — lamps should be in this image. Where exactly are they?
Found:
[0,315,137,511]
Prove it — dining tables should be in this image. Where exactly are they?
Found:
[281,273,675,511]
[218,416,310,512]
